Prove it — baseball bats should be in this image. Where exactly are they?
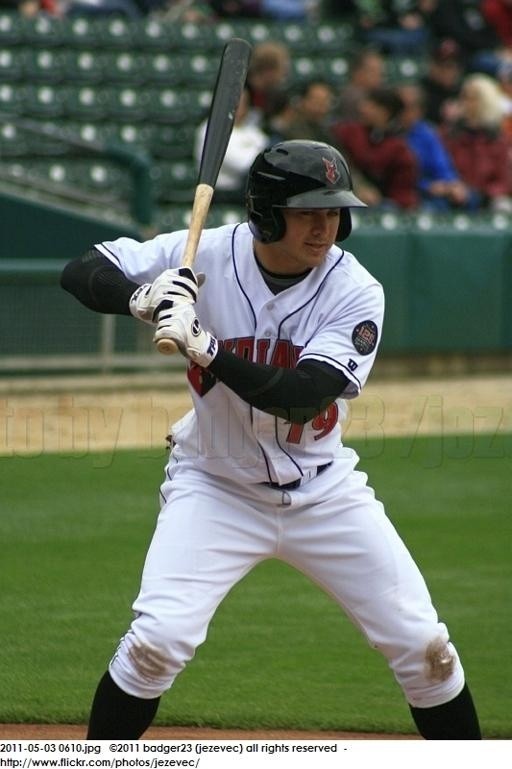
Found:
[158,38,250,354]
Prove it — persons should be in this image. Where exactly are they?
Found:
[59,135,486,739]
[146,0,512,217]
[10,0,162,21]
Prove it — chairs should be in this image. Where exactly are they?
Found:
[0,13,512,231]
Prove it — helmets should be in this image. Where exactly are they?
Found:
[246,139,369,244]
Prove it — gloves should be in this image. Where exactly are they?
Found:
[153,298,219,369]
[128,267,206,325]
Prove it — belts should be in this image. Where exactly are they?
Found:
[261,458,336,490]
